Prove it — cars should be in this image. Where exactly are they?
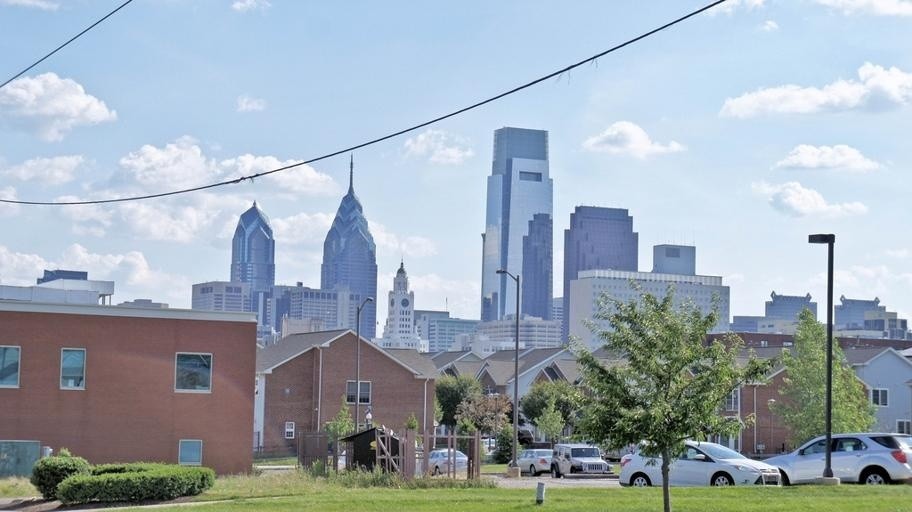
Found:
[428,447,468,477]
[619,439,783,489]
[507,449,553,477]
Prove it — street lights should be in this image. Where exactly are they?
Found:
[804,230,842,488]
[353,294,375,433]
[496,267,521,455]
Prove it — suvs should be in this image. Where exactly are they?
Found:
[760,430,911,488]
[550,442,613,480]
